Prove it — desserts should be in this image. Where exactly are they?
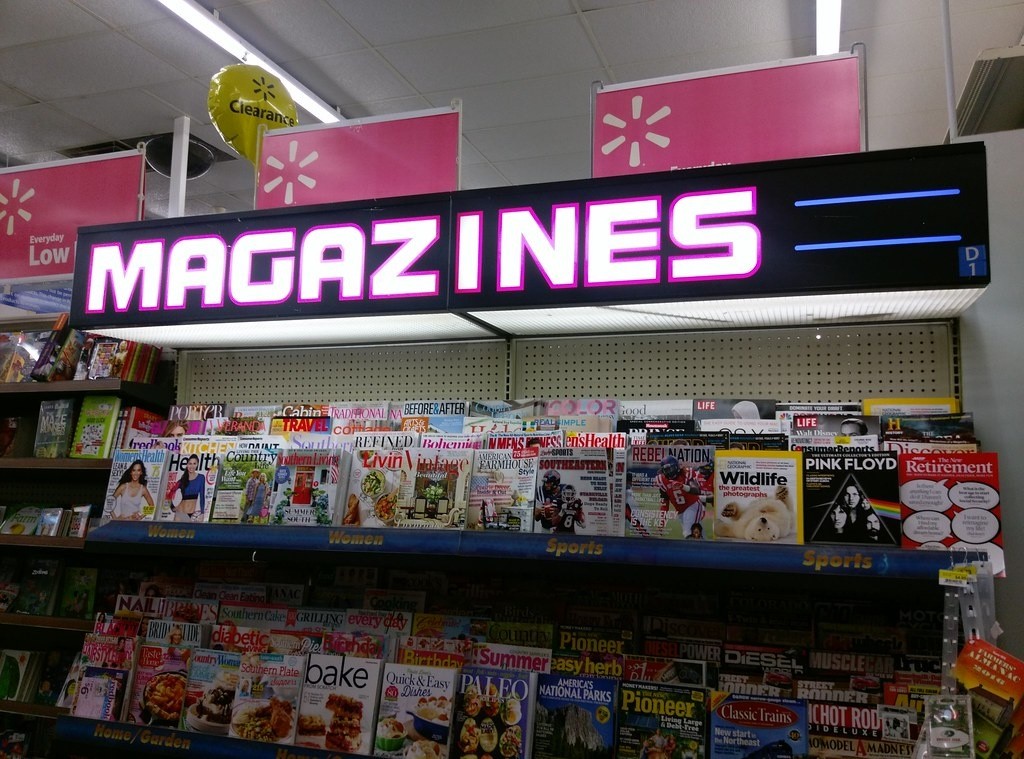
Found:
[717,486,795,541]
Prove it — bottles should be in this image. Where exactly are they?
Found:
[521,498,528,508]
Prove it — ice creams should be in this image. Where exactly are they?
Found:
[408,741,440,759]
[197,686,235,723]
[377,718,405,739]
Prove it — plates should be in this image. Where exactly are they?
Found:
[358,450,375,462]
[229,699,293,742]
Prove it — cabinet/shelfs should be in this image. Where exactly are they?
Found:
[51,320,999,759]
[0,378,161,720]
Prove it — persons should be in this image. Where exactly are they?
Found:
[162,417,189,440]
[111,459,154,522]
[169,455,204,524]
[860,497,871,511]
[842,483,861,524]
[830,502,850,531]
[839,417,868,439]
[731,400,762,421]
[169,626,183,645]
[865,512,882,540]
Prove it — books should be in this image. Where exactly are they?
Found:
[1,332,1024,759]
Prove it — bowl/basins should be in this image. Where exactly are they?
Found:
[376,731,408,751]
[405,705,450,745]
[374,494,396,522]
[361,470,386,498]
